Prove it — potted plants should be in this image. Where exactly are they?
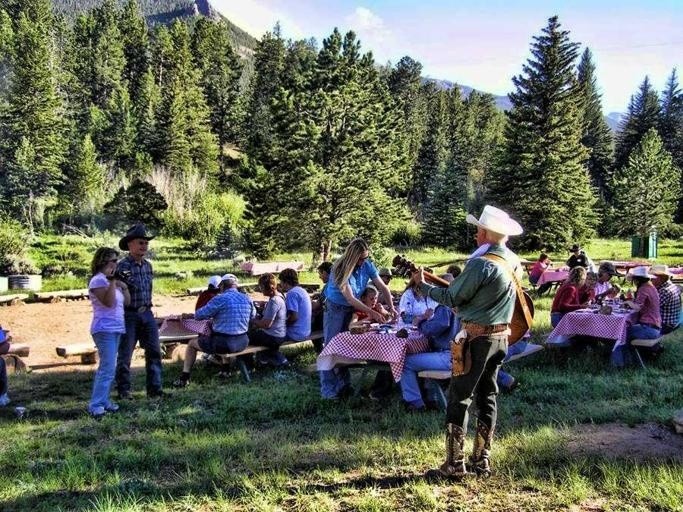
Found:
[2,251,45,292]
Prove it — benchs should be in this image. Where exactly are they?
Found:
[323,313,544,412]
[529,257,683,371]
[56,301,324,384]
[0,343,30,374]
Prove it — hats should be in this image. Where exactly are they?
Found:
[567,242,585,252]
[465,203,524,237]
[598,259,671,281]
[207,273,240,288]
[378,267,393,276]
[118,223,154,251]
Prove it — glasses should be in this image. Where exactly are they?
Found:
[102,257,118,265]
[132,240,151,246]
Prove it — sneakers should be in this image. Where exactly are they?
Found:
[104,400,119,411]
[144,390,162,402]
[117,391,135,400]
[171,377,190,388]
[215,358,289,378]
[86,404,104,416]
[320,383,442,413]
[502,373,518,394]
[0,393,12,406]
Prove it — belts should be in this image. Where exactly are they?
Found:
[641,321,662,331]
[124,304,154,314]
[459,318,511,336]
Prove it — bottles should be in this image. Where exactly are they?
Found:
[604,288,631,313]
[405,302,413,333]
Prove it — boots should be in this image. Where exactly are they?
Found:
[465,417,497,477]
[424,422,467,481]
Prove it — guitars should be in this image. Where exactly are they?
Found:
[392,256,534,348]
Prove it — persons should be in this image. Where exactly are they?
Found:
[1,326,14,407]
[114,226,172,400]
[85,247,132,416]
[411,204,522,483]
